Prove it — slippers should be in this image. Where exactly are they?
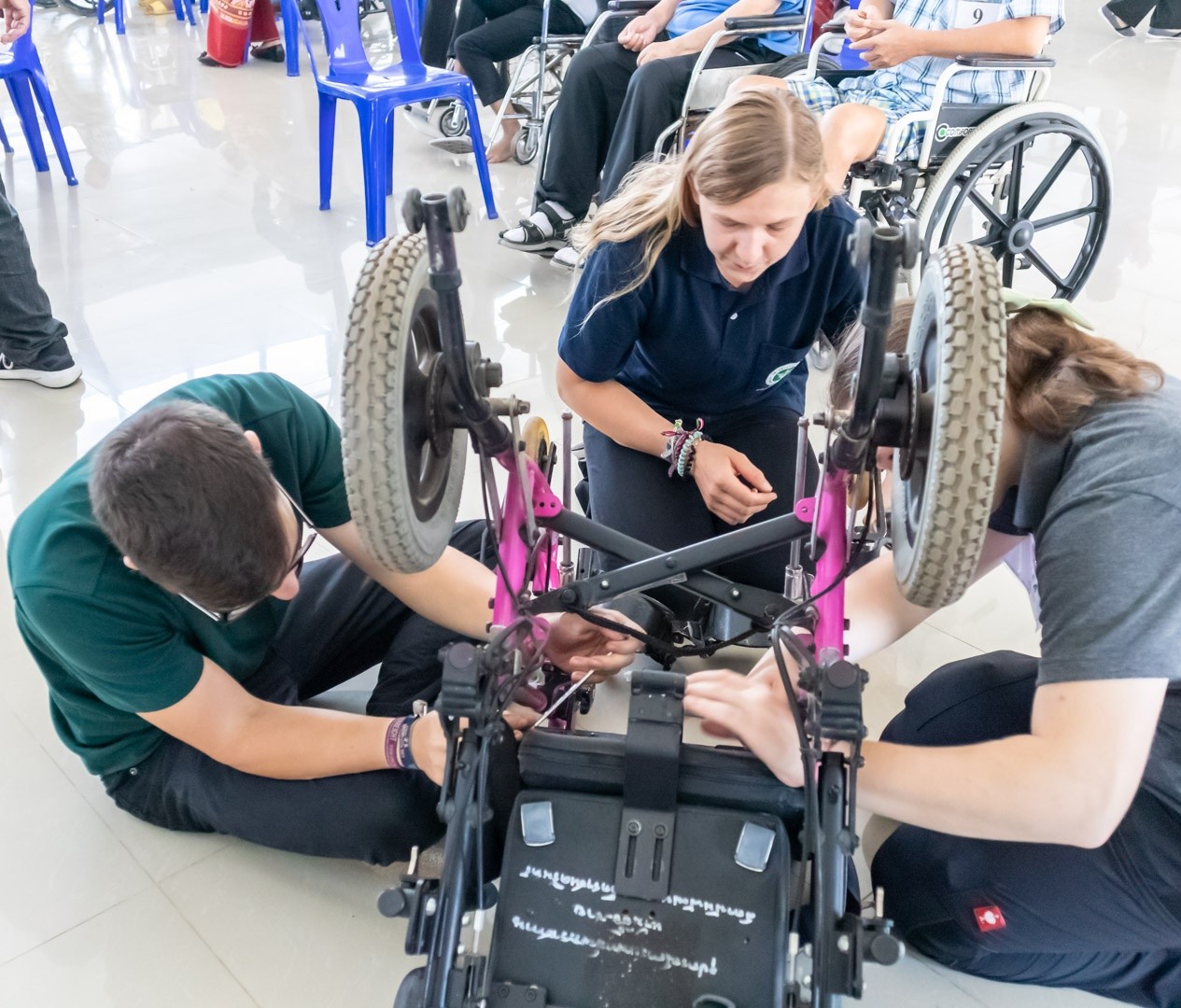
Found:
[137,0,176,15]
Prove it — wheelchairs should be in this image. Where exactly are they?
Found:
[403,0,622,164]
[801,21,1113,306]
[341,188,1009,1008]
[531,0,814,276]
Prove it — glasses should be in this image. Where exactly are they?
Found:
[177,472,318,624]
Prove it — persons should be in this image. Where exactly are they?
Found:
[0,369,651,867]
[406,0,1065,259]
[196,0,287,68]
[554,83,880,615]
[1097,0,1181,40]
[675,285,1181,1008]
[0,0,83,389]
[137,0,176,16]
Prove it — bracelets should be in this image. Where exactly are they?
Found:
[384,699,428,771]
[659,417,713,480]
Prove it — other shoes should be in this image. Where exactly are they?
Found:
[1146,27,1181,40]
[1101,4,1139,38]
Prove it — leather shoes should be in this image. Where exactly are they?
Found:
[250,44,285,62]
[198,52,220,67]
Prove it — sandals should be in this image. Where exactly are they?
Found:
[497,203,576,250]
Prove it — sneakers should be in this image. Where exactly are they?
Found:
[0,339,82,388]
